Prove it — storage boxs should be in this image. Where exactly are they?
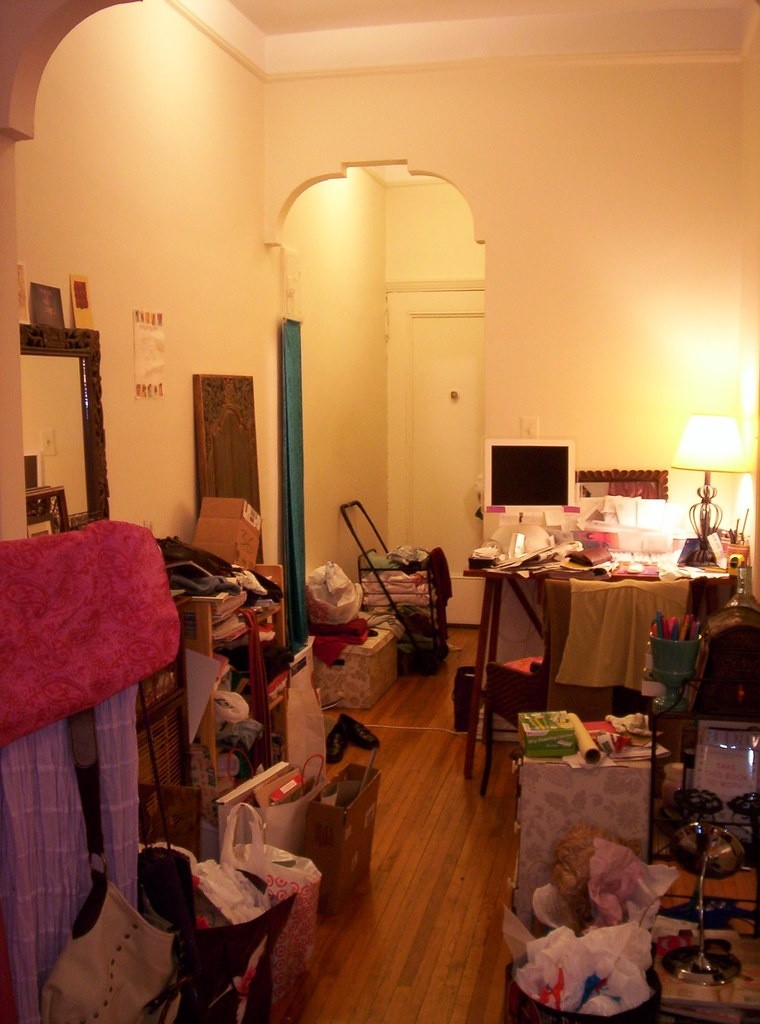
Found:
[701,606,759,722]
[313,629,396,709]
[192,497,263,566]
[518,710,577,757]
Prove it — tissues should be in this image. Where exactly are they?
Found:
[468,546,501,570]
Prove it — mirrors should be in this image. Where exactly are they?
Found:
[19,323,110,538]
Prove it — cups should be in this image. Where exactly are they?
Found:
[726,545,750,573]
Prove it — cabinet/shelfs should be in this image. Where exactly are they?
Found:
[139,565,288,865]
[510,756,652,915]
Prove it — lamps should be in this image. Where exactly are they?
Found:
[671,414,751,567]
[663,821,748,984]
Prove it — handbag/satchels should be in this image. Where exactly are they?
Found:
[39,741,386,1024]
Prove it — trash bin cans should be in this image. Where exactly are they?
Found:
[501,952,663,1024]
[530,885,584,939]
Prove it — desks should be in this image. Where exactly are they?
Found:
[463,562,729,779]
[653,858,759,1024]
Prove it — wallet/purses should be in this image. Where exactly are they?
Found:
[565,546,612,567]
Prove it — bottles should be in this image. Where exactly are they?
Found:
[724,566,760,614]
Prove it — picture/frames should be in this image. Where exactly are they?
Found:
[26,486,70,538]
[31,283,65,325]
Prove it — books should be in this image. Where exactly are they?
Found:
[491,541,661,582]
[582,721,671,762]
[651,915,760,1010]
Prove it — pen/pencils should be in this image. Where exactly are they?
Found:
[729,529,744,545]
[650,610,700,642]
